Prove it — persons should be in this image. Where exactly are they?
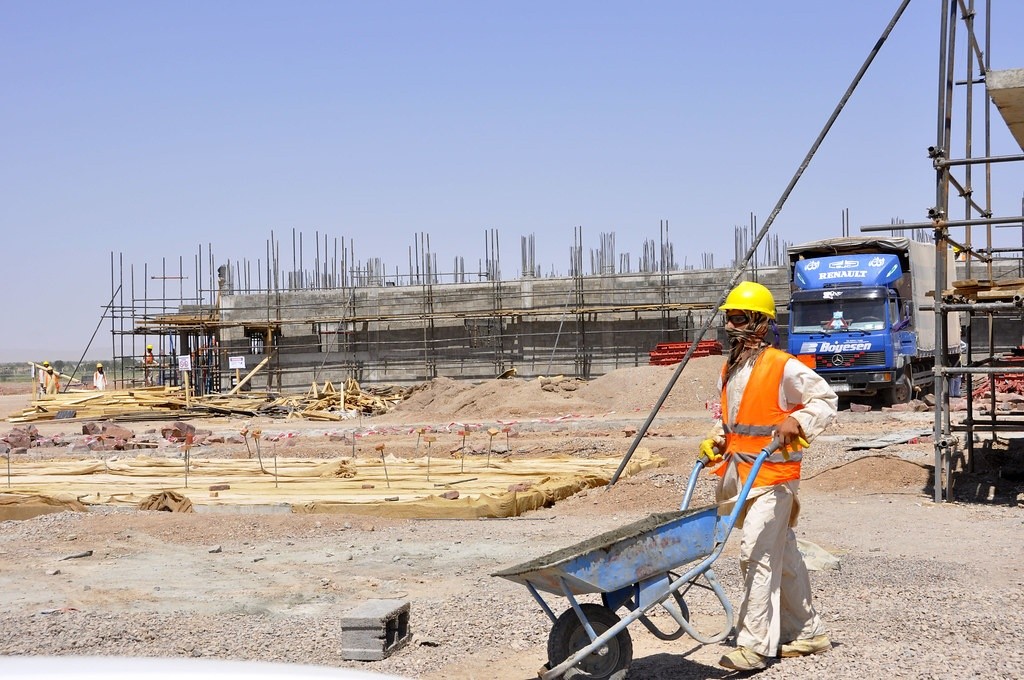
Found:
[947,353,963,398]
[43,366,59,395]
[694,280,838,671]
[39,361,50,394]
[142,345,160,387]
[952,246,966,262]
[93,363,108,391]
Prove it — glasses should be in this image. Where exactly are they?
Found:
[725,313,763,327]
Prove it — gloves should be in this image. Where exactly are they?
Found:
[700,437,724,468]
[770,429,810,460]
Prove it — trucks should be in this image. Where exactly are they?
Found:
[785,235,960,407]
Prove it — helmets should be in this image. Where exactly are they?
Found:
[43,361,49,364]
[146,344,153,349]
[96,363,102,368]
[717,281,776,320]
[953,246,960,253]
[47,366,53,370]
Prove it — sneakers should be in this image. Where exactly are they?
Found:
[718,645,767,670]
[777,635,833,658]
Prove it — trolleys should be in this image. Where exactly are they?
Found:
[491,437,780,678]
